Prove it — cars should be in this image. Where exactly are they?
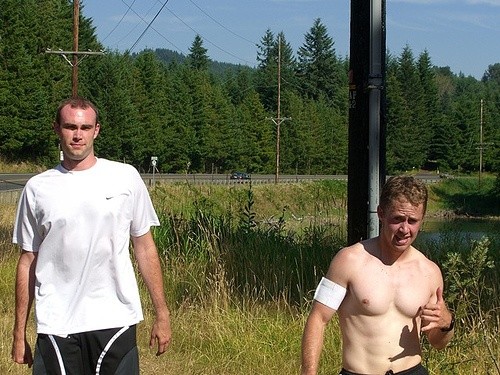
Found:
[230,172,251,183]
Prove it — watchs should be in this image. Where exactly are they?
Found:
[440,314,456,333]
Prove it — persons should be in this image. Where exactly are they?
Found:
[300,175,454,375]
[12,98,172,375]
